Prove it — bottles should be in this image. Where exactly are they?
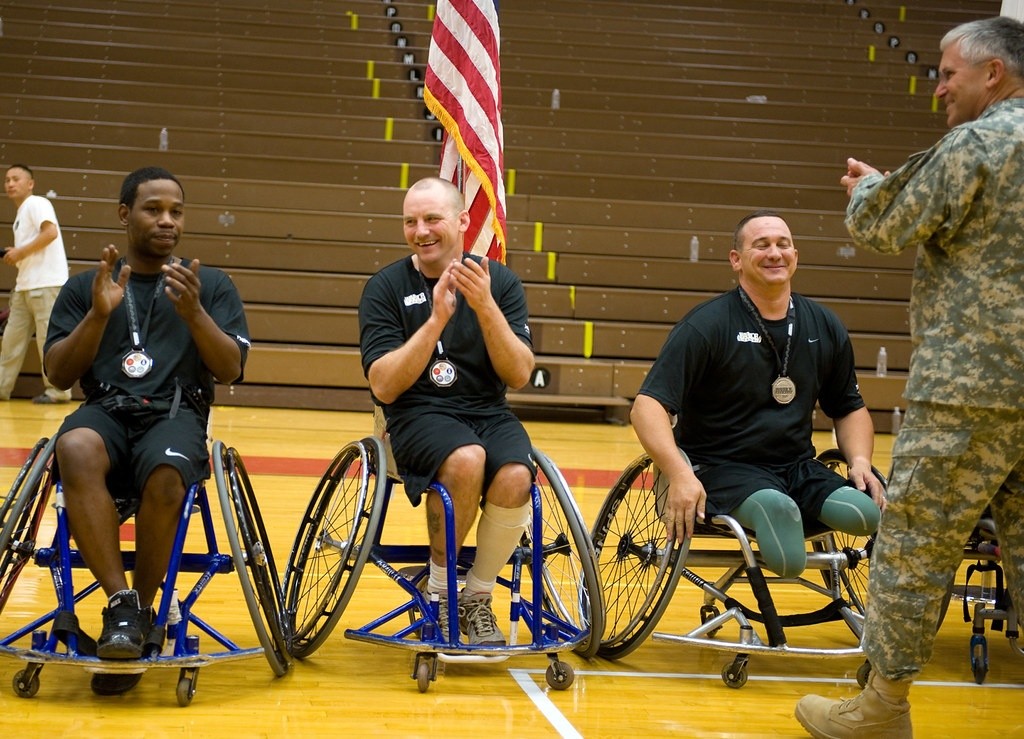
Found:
[746,94,768,103]
[159,127,168,151]
[690,236,699,262]
[552,89,559,107]
[832,427,838,445]
[855,384,861,395]
[876,347,887,376]
[892,406,900,435]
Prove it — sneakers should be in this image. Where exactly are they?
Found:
[91,606,169,696]
[458,586,507,647]
[424,584,466,643]
[792,686,911,739]
[95,589,151,660]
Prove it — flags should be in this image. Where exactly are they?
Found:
[423,3,509,270]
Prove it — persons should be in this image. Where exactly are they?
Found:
[794,16,1024,737]
[43,165,250,695]
[358,176,538,656]
[630,214,887,578]
[0,164,74,403]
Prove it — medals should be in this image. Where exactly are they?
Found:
[771,375,797,404]
[119,349,154,378]
[428,357,460,387]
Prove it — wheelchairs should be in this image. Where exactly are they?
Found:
[0,432,292,706]
[281,403,607,693]
[578,411,1024,689]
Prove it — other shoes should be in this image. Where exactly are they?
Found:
[32,391,68,405]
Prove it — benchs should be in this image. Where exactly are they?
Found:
[0,0,1003,431]
